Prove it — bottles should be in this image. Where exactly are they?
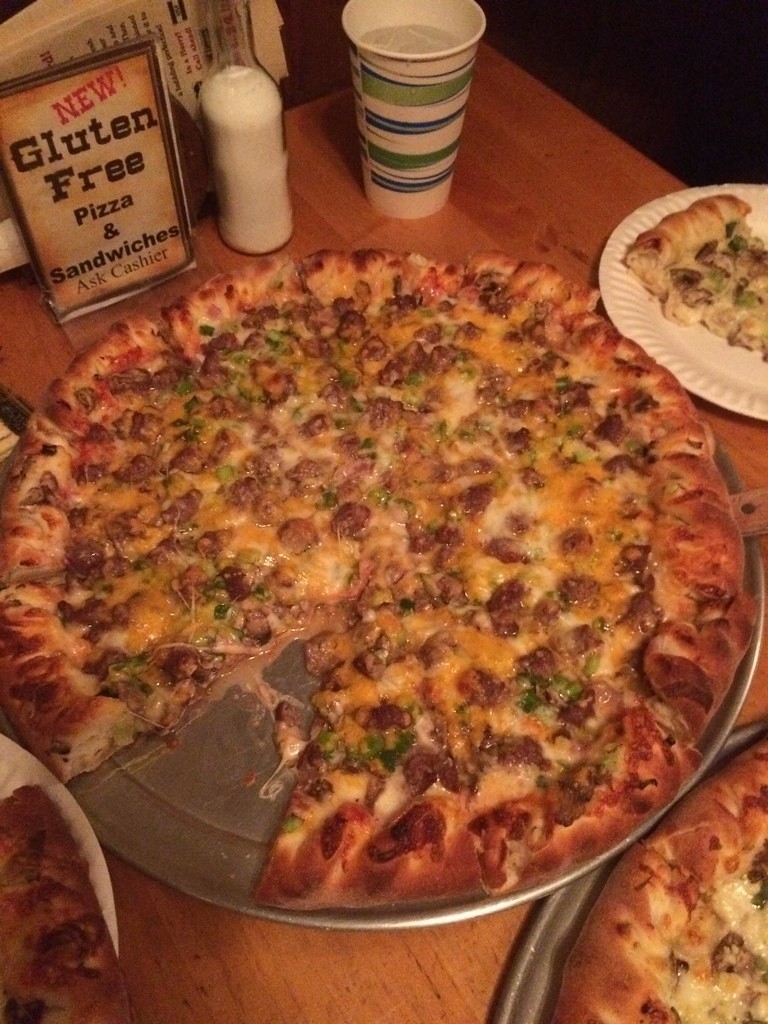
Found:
[197,0,293,257]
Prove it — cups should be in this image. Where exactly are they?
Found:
[341,0,487,221]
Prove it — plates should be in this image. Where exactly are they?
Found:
[0,733,120,956]
[69,447,766,930]
[499,722,768,1024]
[599,182,767,423]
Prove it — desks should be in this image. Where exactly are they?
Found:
[0,0,768,1024]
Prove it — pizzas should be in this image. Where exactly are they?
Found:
[0,786,133,1024]
[552,740,768,1024]
[0,249,756,906]
[622,194,768,357]
